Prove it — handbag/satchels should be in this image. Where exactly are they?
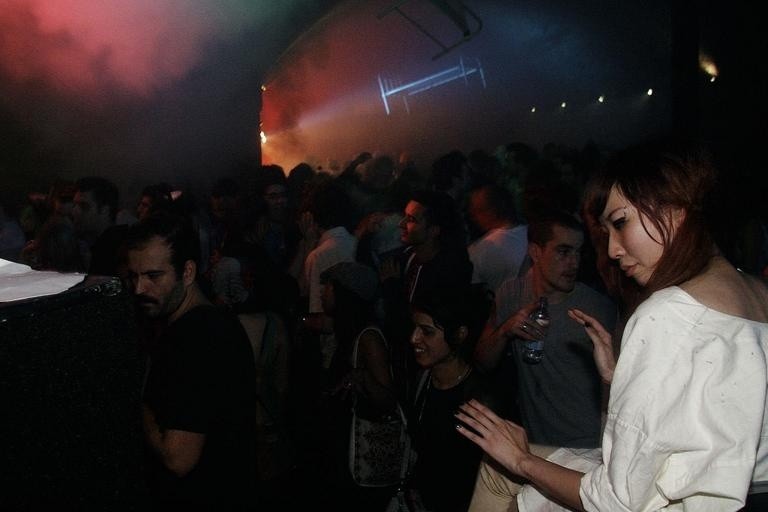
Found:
[344,328,413,488]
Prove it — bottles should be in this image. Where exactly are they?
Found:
[520,296,551,364]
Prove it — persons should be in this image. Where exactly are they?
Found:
[451,141,768,511]
[0,141,639,512]
